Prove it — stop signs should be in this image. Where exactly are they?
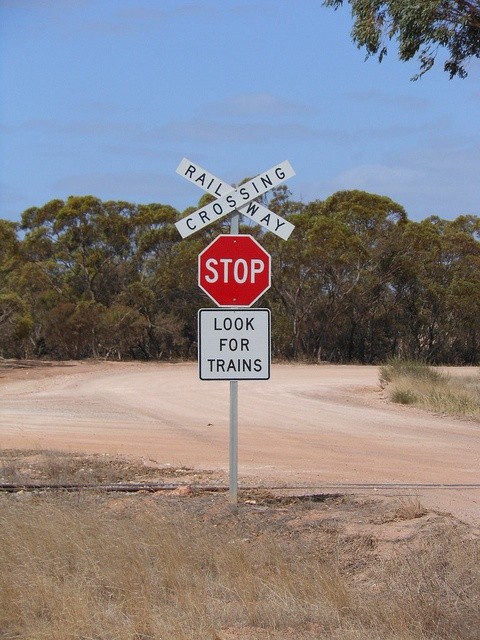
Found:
[199,235,272,307]
